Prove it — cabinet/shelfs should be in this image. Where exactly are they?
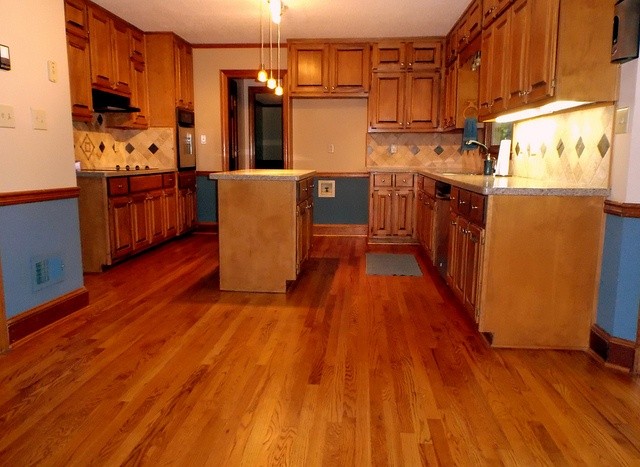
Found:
[445,187,607,350]
[129,175,165,255]
[78,173,130,272]
[104,24,148,127]
[287,40,369,97]
[443,0,481,133]
[370,172,419,242]
[162,171,178,240]
[87,1,131,99]
[479,1,620,122]
[177,172,197,234]
[65,0,93,122]
[369,40,443,132]
[419,176,438,265]
[209,169,317,293]
[148,32,195,110]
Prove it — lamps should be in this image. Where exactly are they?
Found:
[275,24,284,97]
[257,1,269,82]
[266,1,277,90]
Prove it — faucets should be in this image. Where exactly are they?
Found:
[465,140,489,154]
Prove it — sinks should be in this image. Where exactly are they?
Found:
[425,167,481,175]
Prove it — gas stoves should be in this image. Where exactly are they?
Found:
[97,164,154,170]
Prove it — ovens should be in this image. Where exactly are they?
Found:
[176,110,196,171]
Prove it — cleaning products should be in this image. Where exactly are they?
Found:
[484,153,492,175]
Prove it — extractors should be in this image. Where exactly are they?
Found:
[92,88,141,113]
